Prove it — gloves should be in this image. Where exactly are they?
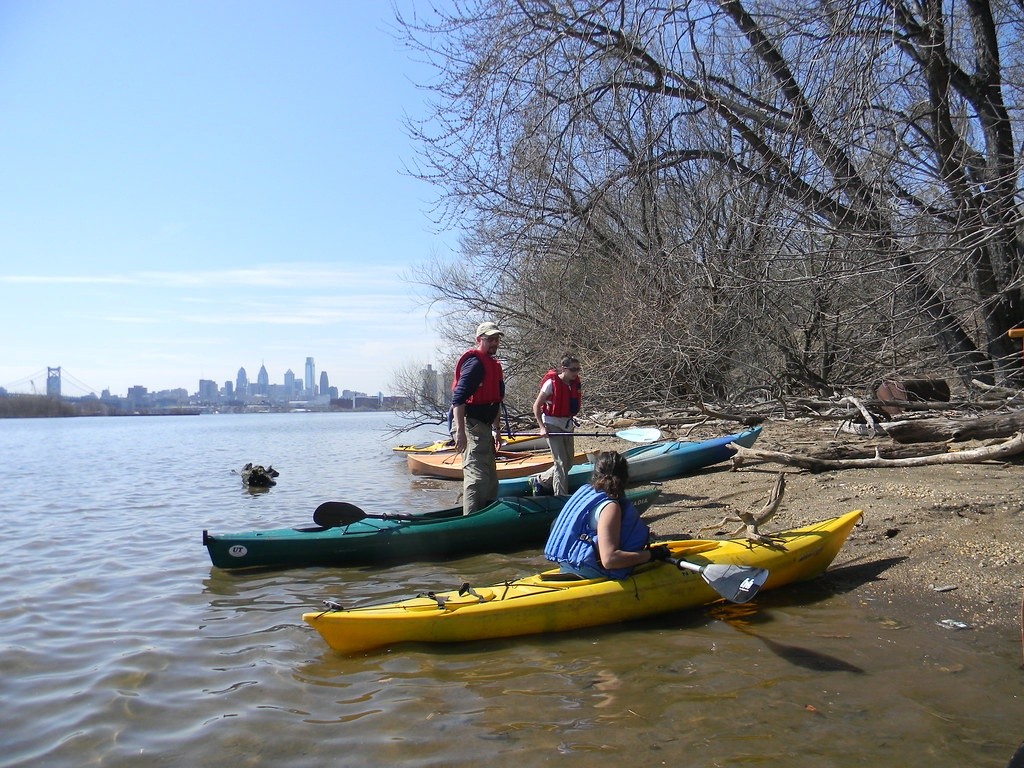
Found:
[649,545,671,562]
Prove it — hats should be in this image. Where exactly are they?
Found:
[476,322,504,337]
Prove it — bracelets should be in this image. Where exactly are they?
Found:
[492,427,500,431]
[528,354,581,496]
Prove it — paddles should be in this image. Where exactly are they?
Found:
[312,499,425,527]
[492,427,664,444]
[662,554,770,604]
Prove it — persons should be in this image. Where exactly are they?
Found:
[544,450,672,578]
[448,322,515,516]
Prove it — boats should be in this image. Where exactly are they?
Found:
[497,426,762,498]
[301,509,864,653]
[407,450,600,479]
[202,481,662,569]
[392,435,550,457]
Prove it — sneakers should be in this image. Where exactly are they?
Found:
[529,476,544,497]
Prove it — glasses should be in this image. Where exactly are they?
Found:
[481,336,501,342]
[564,367,580,372]
